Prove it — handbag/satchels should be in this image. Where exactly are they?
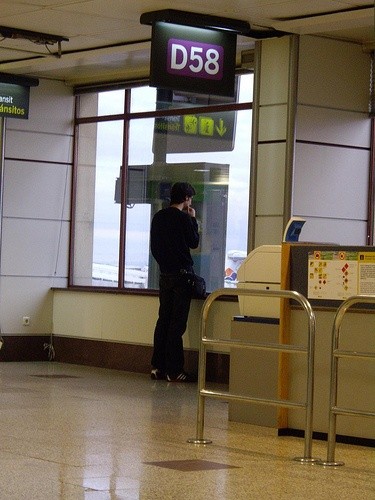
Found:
[179,271,207,297]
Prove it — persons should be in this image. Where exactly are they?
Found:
[150,182,200,383]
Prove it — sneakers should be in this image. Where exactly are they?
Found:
[150,369,166,379]
[167,371,195,382]
